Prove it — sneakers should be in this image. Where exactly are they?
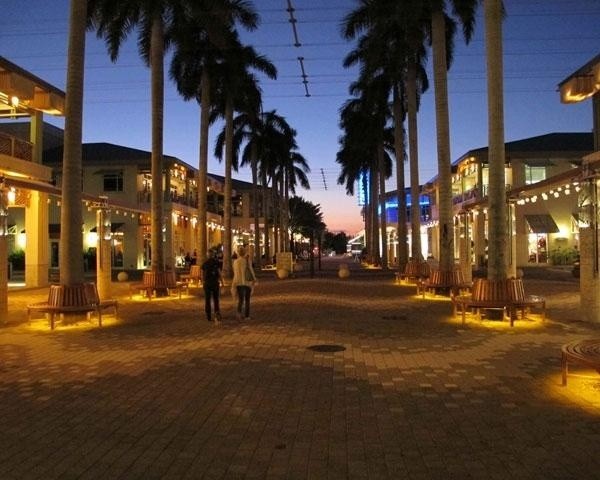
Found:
[207,316,213,321]
[245,317,251,320]
[215,313,221,320]
[238,313,242,318]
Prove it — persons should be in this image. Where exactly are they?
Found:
[201,248,224,321]
[230,247,257,321]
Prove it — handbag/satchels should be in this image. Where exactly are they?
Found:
[245,268,254,281]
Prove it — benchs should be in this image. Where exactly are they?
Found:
[560,337,600,387]
[394,271,462,299]
[129,274,204,301]
[450,290,546,327]
[26,298,118,332]
